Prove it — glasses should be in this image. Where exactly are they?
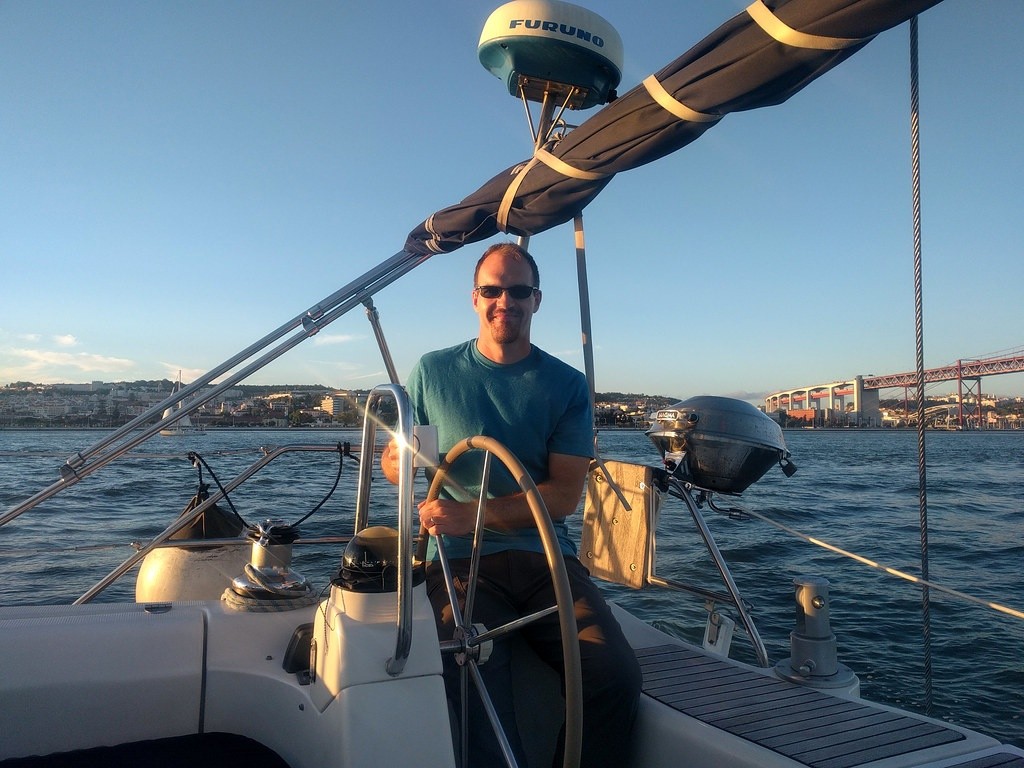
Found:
[476,285,539,299]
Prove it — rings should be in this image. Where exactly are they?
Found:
[431,516,435,525]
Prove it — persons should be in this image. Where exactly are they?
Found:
[380,245,643,768]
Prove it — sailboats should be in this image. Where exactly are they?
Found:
[159,369,208,437]
